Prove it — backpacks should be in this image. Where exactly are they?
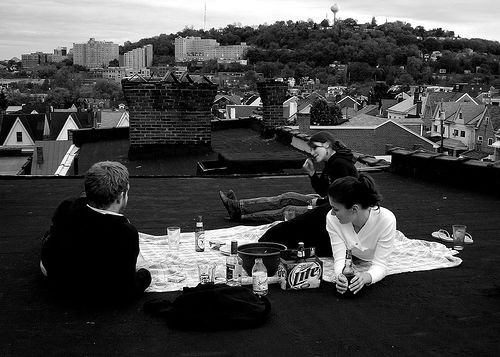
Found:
[163,282,272,333]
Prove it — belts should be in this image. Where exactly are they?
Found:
[40,260,48,277]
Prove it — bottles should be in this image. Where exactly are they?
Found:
[341,251,355,300]
[306,200,313,213]
[225,241,244,288]
[251,258,269,298]
[195,216,204,252]
[297,242,306,264]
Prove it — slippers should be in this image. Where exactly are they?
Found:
[432,229,453,243]
[452,232,474,245]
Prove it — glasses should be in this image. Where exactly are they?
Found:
[311,144,324,151]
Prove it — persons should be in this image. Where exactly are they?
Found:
[258,174,396,294]
[220,131,358,222]
[40,161,150,313]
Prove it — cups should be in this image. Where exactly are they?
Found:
[284,210,294,221]
[166,228,180,250]
[196,261,217,285]
[451,224,466,251]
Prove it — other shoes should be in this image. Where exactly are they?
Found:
[220,190,241,219]
[227,190,237,200]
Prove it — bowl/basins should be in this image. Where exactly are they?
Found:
[238,240,288,277]
